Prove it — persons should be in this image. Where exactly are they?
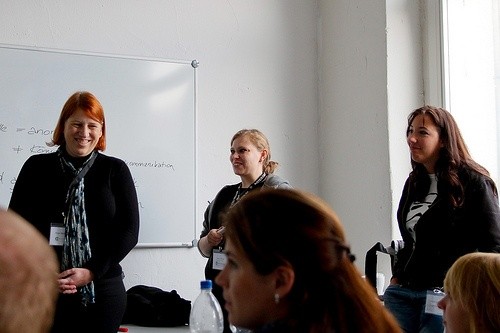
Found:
[8,91,140,333]
[437,252,500,333]
[384,106,500,333]
[197,129,290,333]
[0,209,59,333]
[216,187,401,333]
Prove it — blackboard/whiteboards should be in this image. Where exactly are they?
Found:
[0,43,198,248]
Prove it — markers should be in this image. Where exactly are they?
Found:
[119,327,128,332]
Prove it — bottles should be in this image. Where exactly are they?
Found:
[189,280,225,333]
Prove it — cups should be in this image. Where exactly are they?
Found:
[229,323,252,333]
